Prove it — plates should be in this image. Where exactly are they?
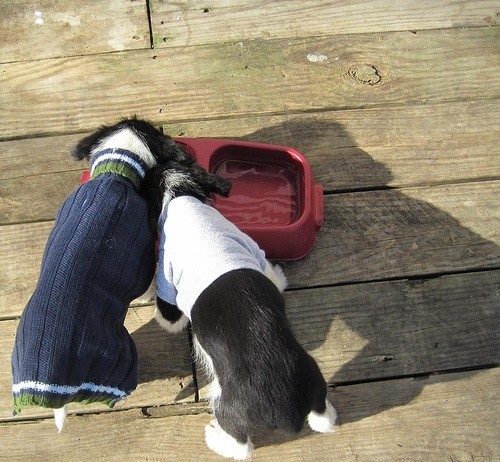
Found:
[82,137,325,262]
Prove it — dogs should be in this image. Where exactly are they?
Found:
[10,115,187,435]
[140,161,338,462]
